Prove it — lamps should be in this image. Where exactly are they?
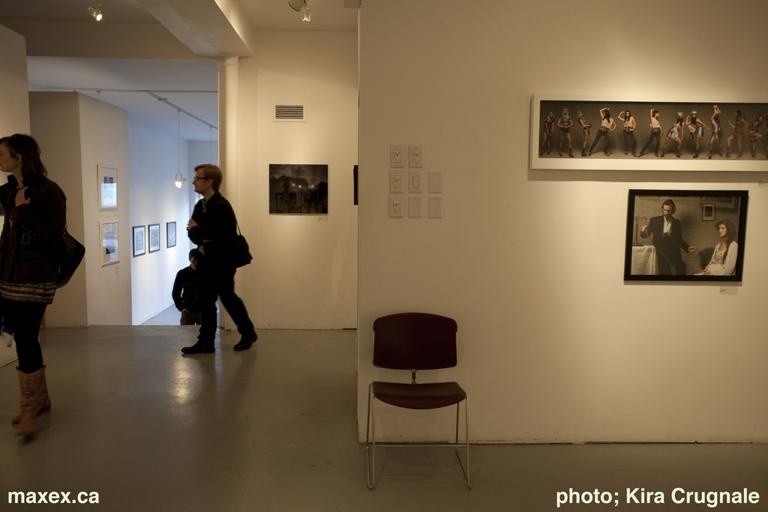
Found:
[84,2,111,22]
[289,0,312,25]
[174,109,187,191]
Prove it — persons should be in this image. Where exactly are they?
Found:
[640,198,696,276]
[172,247,207,325]
[700,219,740,276]
[0,133,68,439]
[541,103,765,159]
[179,162,260,356]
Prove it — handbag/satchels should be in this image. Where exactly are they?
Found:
[204,234,253,270]
[46,230,86,284]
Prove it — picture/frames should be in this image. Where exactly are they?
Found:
[98,219,121,268]
[621,186,749,283]
[166,221,177,248]
[132,225,146,257]
[147,223,161,254]
[526,85,763,179]
[96,160,119,214]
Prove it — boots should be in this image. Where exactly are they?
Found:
[12,366,51,436]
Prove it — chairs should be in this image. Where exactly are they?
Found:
[361,311,476,490]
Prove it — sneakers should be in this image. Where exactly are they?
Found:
[234,331,257,351]
[182,338,215,354]
